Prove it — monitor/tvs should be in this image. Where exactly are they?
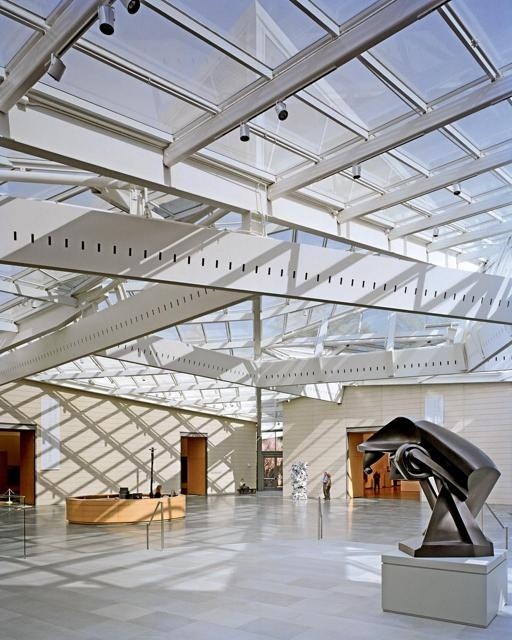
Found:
[132,493,142,499]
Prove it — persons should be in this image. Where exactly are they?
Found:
[249,478,259,495]
[322,472,329,499]
[372,471,381,491]
[325,473,331,501]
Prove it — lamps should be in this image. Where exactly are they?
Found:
[453,183,460,195]
[433,228,439,238]
[274,100,289,122]
[95,1,143,37]
[44,52,66,83]
[352,161,361,180]
[238,121,250,142]
